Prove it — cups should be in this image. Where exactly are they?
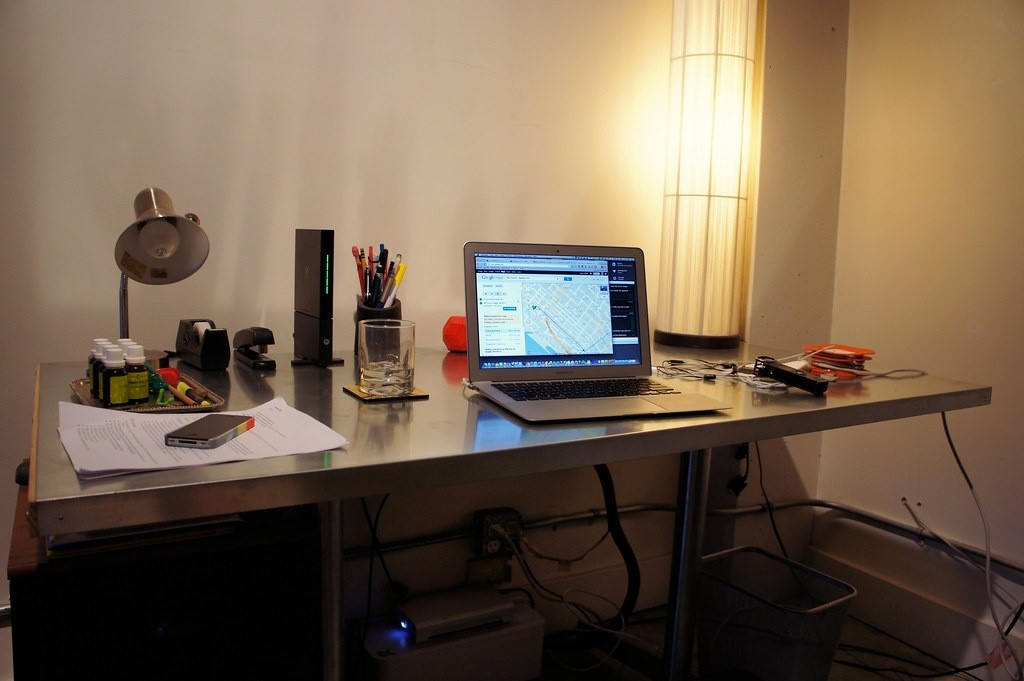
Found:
[353,297,402,355]
[358,319,416,396]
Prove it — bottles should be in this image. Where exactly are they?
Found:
[88,335,149,408]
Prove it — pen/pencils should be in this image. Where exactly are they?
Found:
[352,243,407,308]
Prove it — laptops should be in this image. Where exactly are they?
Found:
[463,241,734,422]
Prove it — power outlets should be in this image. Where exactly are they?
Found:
[472,507,522,557]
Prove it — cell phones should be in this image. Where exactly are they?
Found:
[164,413,255,449]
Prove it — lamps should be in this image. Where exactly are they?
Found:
[653,0,767,340]
[113,187,210,369]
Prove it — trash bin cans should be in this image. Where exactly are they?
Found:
[694,544,857,681]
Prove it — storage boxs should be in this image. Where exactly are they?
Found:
[696,546,859,681]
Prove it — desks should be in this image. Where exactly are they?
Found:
[26,341,993,681]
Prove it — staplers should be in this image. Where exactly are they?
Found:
[233,326,276,370]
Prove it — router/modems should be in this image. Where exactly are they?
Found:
[393,582,516,646]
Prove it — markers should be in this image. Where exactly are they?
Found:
[176,381,210,406]
